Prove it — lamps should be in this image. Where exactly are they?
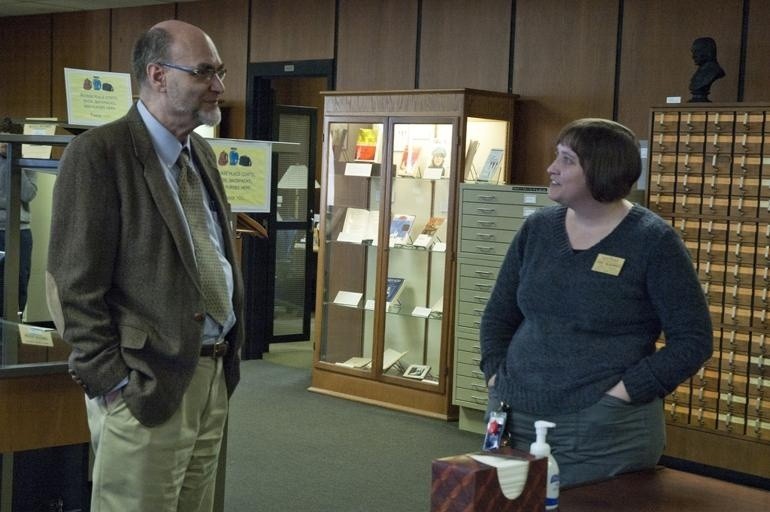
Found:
[276,164,321,219]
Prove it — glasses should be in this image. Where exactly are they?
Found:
[159,62,225,79]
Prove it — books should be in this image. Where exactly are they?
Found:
[336,207,447,250]
[333,277,444,317]
[344,127,452,180]
[335,347,432,380]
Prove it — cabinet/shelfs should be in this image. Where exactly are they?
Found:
[309,88,520,422]
[451,183,645,433]
[644,101,770,481]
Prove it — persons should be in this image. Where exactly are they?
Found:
[46,19,245,512]
[689,37,726,91]
[0,113,38,324]
[480,117,714,491]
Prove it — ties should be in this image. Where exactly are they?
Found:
[177,152,231,325]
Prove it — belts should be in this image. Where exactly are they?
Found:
[199,343,229,357]
